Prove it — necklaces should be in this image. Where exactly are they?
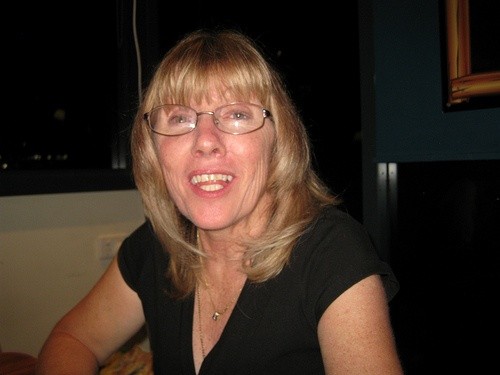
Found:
[194,280,208,361]
[197,227,245,321]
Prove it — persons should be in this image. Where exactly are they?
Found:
[36,35,403,375]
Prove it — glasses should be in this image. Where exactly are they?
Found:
[144,101,273,136]
